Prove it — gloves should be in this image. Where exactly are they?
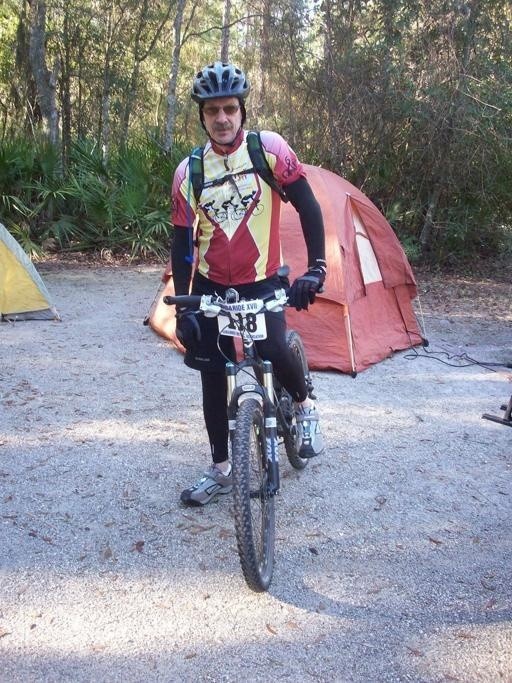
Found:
[288,273,325,311]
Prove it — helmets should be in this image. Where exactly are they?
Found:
[190,62,250,103]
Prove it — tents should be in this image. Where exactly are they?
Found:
[0,223,63,322]
[144,162,429,378]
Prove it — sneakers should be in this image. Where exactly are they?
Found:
[295,397,323,457]
[180,462,232,505]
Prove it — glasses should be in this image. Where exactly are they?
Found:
[201,106,238,113]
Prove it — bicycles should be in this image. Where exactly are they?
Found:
[163,287,323,591]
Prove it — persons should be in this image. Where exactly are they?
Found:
[169,60,327,509]
